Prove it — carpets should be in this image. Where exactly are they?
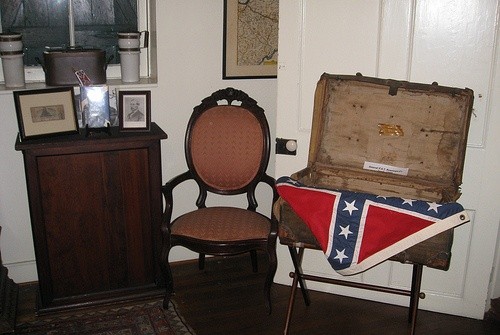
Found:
[13,296,195,335]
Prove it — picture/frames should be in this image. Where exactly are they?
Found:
[120,89,153,132]
[13,87,80,143]
[223,0,279,80]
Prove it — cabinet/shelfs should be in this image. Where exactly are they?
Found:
[11,122,171,315]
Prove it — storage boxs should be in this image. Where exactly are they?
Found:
[275,71,475,272]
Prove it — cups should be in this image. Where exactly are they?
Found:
[117,30,140,83]
[0,32,25,88]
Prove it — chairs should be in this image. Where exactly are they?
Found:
[150,86,284,313]
